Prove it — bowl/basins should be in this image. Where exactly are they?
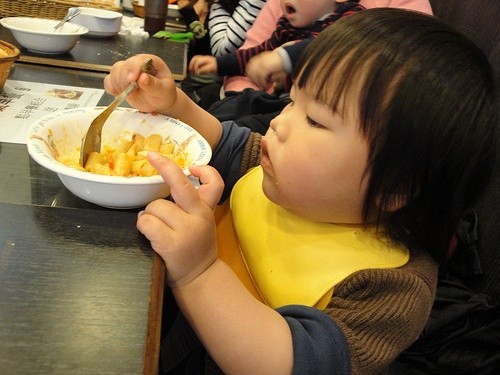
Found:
[0,39,21,92]
[68,7,123,38]
[0,17,90,54]
[27,106,212,210]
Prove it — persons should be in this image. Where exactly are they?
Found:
[181,0,435,111]
[186,0,368,135]
[103,7,500,375]
[174,0,266,59]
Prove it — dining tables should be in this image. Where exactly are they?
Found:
[0,0,186,375]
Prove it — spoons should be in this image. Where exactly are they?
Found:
[79,57,159,168]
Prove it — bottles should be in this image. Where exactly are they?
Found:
[144,0,169,32]
